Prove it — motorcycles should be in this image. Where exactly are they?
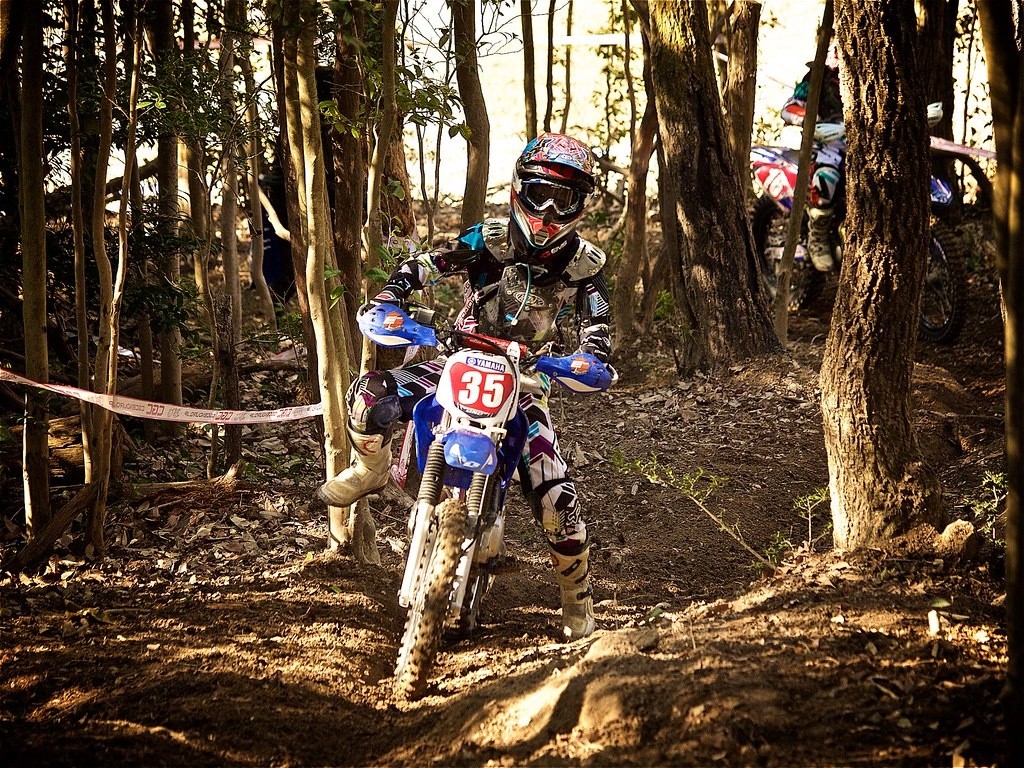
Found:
[350,302,620,702]
[751,100,968,346]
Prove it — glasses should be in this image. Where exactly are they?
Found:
[520,178,580,215]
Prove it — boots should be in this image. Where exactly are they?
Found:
[551,541,596,640]
[805,207,833,272]
[317,423,390,506]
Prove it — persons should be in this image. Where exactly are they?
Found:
[317,135,618,643]
[751,56,846,271]
[248,65,340,303]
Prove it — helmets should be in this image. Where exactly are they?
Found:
[512,135,593,250]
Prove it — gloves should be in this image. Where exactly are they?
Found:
[576,335,612,377]
[361,287,403,325]
